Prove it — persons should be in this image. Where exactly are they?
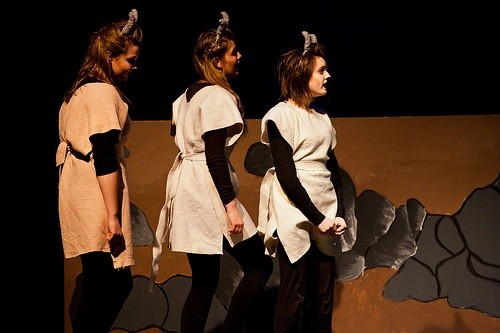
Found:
[54,8,142,333]
[151,12,275,332]
[256,30,357,333]
